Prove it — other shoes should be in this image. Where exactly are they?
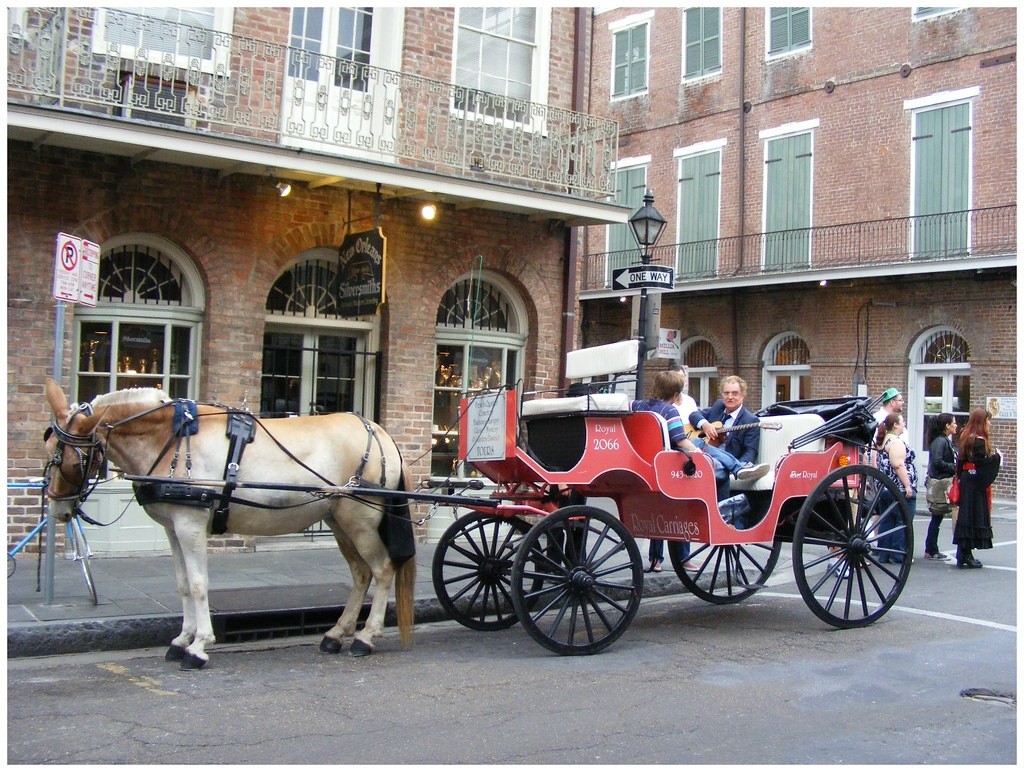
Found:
[650,559,661,571]
[682,561,700,571]
[872,549,878,557]
[893,556,915,564]
[737,462,770,480]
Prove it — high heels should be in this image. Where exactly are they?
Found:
[957,555,983,569]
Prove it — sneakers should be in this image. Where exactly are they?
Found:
[925,551,951,561]
[826,562,850,578]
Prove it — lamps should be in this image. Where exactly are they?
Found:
[265,165,291,197]
[423,194,442,221]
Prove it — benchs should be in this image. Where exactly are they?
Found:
[623,408,825,493]
[518,336,647,422]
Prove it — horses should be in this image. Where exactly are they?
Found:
[44,378,417,671]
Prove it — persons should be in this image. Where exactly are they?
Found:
[871,387,909,467]
[924,413,959,561]
[628,371,770,501]
[952,407,1002,569]
[648,364,700,572]
[688,375,761,467]
[546,383,592,566]
[826,547,871,578]
[876,413,918,564]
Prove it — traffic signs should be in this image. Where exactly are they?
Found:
[610,265,676,292]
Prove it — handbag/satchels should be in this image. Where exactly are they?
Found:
[946,474,960,505]
[925,477,953,516]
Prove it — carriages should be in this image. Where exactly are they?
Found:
[39,369,919,676]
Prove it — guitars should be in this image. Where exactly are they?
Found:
[683,419,783,448]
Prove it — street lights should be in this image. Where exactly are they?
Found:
[624,189,667,402]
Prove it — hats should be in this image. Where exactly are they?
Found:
[882,387,902,404]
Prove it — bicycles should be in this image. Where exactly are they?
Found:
[7,478,99,606]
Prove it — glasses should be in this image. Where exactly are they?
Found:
[893,398,903,402]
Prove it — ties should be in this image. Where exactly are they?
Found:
[722,413,731,425]
